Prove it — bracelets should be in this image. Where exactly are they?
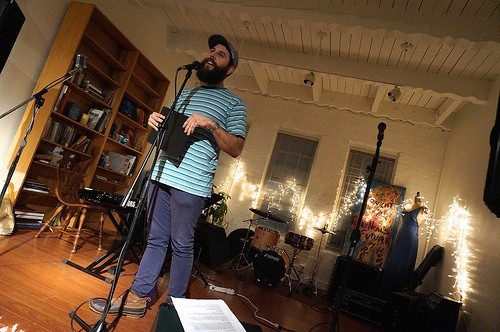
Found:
[208,122,219,131]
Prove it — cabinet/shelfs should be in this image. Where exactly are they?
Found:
[1,1,172,231]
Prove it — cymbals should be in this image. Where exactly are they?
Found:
[249,208,287,223]
[314,227,336,235]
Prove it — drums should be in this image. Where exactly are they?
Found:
[253,246,290,283]
[250,225,281,253]
[284,231,314,251]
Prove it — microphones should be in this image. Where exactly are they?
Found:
[65,54,86,77]
[177,61,202,71]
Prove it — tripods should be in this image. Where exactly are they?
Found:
[229,216,268,272]
[279,231,324,298]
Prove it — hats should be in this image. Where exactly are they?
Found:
[208,34,239,69]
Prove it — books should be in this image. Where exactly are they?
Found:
[43,117,90,152]
[23,181,49,194]
[111,122,139,148]
[54,85,111,135]
[35,154,63,160]
[119,97,147,128]
[13,205,45,229]
[74,66,114,105]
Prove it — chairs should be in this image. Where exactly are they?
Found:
[34,159,106,254]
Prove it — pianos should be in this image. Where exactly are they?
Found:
[61,187,146,284]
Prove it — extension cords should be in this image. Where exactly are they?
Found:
[209,285,234,294]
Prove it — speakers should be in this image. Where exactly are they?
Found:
[0,0,26,74]
[326,255,383,305]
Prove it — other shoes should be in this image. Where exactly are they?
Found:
[88,289,152,319]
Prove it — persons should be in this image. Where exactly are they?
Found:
[89,34,247,317]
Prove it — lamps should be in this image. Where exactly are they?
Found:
[304,30,329,87]
[387,43,415,102]
